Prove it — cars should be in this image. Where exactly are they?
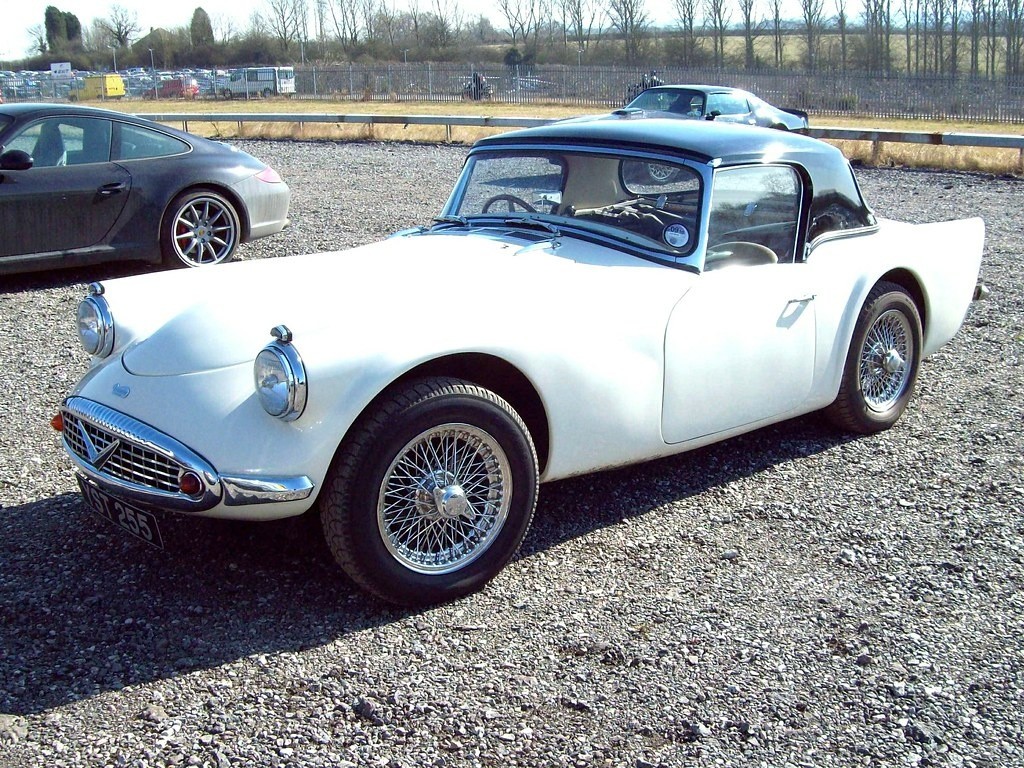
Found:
[47,106,987,609]
[0,67,298,104]
[610,81,806,136]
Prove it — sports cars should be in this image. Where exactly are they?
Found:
[0,100,292,278]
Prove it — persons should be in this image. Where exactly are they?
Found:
[0,88,3,104]
[635,69,665,92]
[467,72,484,100]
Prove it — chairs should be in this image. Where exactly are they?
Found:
[30,123,66,167]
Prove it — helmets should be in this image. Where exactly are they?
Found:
[652,70,656,75]
[642,74,647,77]
[474,72,478,77]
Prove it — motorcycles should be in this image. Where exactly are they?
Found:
[460,82,493,101]
